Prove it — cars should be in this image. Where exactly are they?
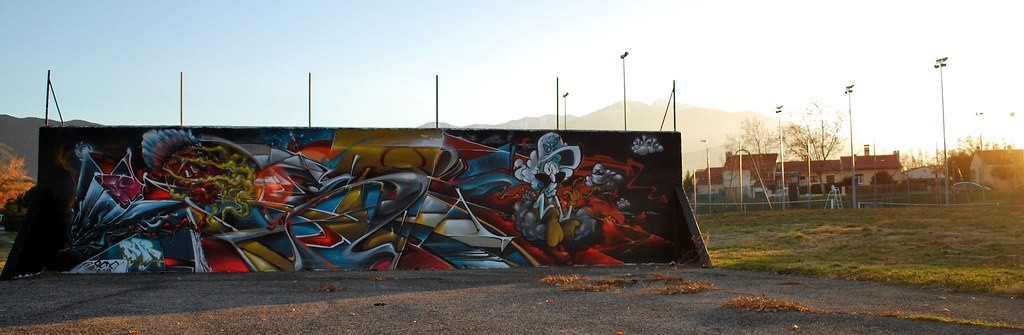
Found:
[950,182,990,194]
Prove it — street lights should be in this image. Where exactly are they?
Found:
[700,139,712,214]
[621,51,630,131]
[976,112,985,182]
[563,92,569,131]
[845,85,859,208]
[934,57,950,207]
[776,105,788,210]
